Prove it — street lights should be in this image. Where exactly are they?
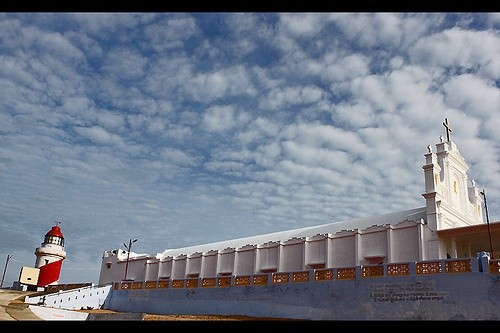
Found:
[480,189,494,260]
[123,237,139,280]
[0,253,14,287]
